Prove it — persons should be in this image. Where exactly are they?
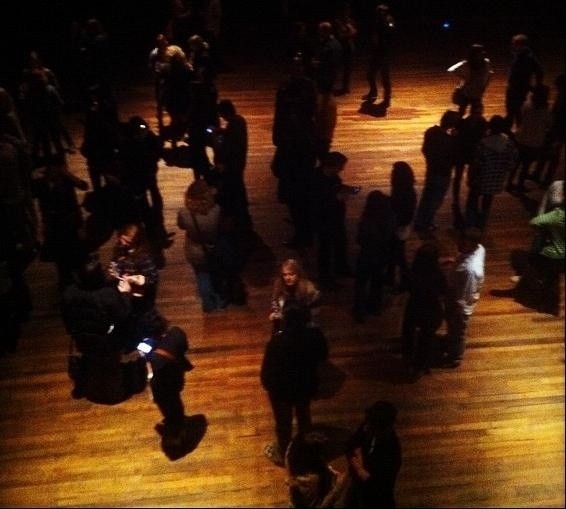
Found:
[363,6,396,102]
[416,110,463,233]
[104,223,159,299]
[1,4,155,225]
[213,99,251,215]
[269,257,321,332]
[338,15,357,93]
[309,76,338,161]
[190,34,219,125]
[304,152,351,292]
[466,116,518,220]
[503,34,544,129]
[177,181,227,314]
[507,84,554,194]
[354,190,401,322]
[203,154,249,305]
[147,34,171,130]
[271,57,316,147]
[284,423,354,507]
[509,178,566,286]
[392,161,417,225]
[309,20,342,91]
[530,204,566,268]
[280,160,318,249]
[159,45,194,150]
[447,45,497,117]
[346,400,401,509]
[261,301,330,467]
[139,310,192,430]
[440,225,486,369]
[401,242,449,376]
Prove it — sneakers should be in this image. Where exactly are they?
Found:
[265,444,285,466]
